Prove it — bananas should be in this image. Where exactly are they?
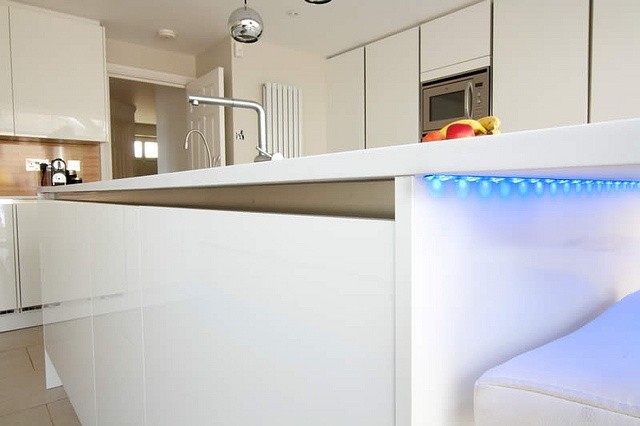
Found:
[441,119,488,135]
[477,116,499,134]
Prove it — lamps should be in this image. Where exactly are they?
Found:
[228,1,263,43]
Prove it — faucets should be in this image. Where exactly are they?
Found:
[189,95,284,162]
[183,129,212,168]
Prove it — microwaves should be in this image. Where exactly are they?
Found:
[421,72,489,134]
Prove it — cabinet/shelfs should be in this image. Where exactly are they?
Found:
[0,196,43,334]
[490,1,639,133]
[328,27,420,153]
[1,0,110,144]
[420,0,492,82]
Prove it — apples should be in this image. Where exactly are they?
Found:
[422,132,445,142]
[446,124,475,139]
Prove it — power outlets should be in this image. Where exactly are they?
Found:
[25,158,49,171]
[67,159,81,172]
